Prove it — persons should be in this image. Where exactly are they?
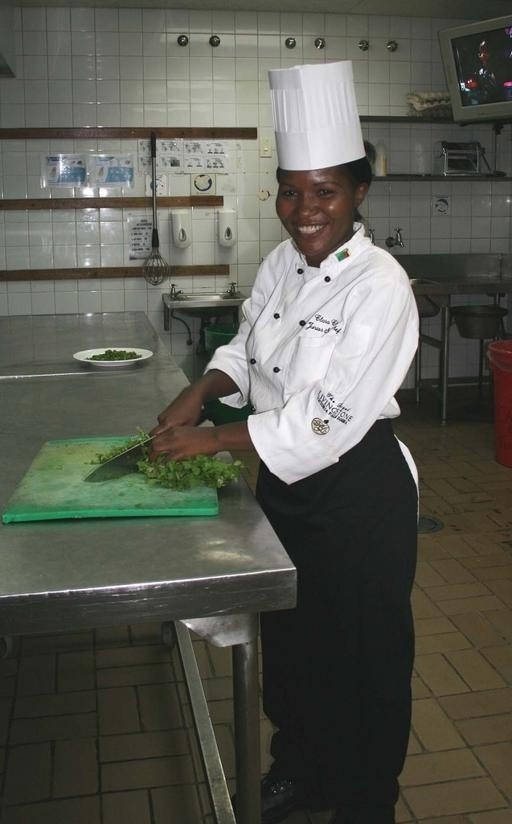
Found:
[147,147,422,824]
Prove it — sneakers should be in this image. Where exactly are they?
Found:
[230,773,333,823]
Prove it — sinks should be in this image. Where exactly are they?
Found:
[162,293,249,318]
[409,277,442,318]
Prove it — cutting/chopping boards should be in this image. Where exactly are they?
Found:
[1,438,218,525]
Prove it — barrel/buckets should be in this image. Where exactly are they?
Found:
[204,323,253,424]
[487,338,512,470]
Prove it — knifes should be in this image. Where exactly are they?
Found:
[82,407,220,483]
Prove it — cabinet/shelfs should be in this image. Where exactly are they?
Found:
[361,114,507,181]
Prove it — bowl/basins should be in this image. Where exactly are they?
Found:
[450,304,509,340]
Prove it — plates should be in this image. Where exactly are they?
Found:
[71,348,154,367]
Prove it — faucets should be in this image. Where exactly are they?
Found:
[368,228,375,244]
[386,227,405,249]
[170,284,183,299]
[224,281,237,296]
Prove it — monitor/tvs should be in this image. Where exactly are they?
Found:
[438,14,512,123]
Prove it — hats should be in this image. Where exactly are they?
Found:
[266,60,367,172]
